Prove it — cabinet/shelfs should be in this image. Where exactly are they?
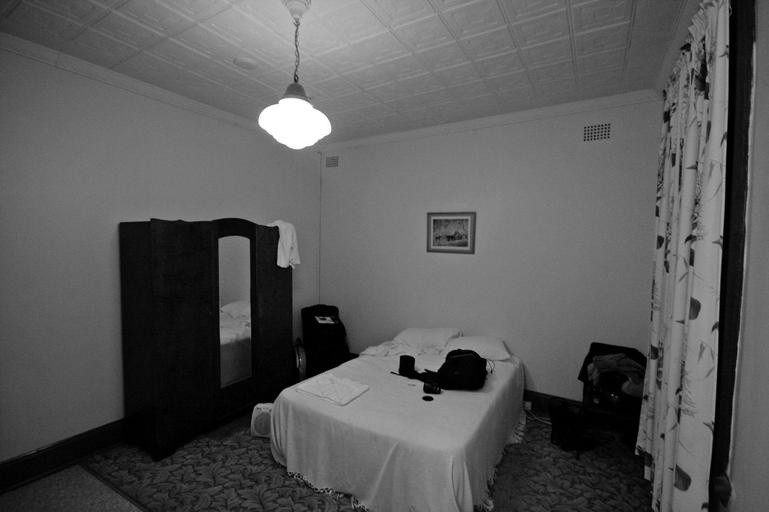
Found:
[118,217,294,462]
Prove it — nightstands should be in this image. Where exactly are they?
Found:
[579,342,646,456]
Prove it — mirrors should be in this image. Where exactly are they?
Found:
[217,233,253,388]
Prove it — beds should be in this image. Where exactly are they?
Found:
[267,336,528,512]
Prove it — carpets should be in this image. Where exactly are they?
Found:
[73,365,652,512]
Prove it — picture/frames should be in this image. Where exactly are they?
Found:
[426,210,476,255]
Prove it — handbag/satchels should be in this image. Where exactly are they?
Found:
[438,349,487,390]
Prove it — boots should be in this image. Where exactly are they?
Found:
[549,397,602,452]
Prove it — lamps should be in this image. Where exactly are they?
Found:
[255,2,333,155]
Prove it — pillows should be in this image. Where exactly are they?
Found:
[395,325,511,364]
